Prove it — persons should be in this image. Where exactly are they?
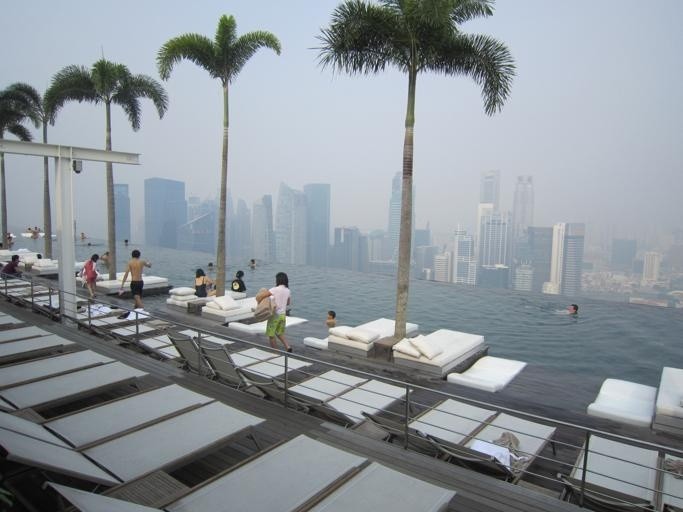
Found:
[232,271,246,292]
[121,250,151,308]
[258,272,292,353]
[569,303,578,316]
[102,251,109,261]
[0,255,23,279]
[125,239,128,246]
[248,259,256,269]
[0,227,40,250]
[195,269,212,297]
[326,310,336,327]
[80,254,99,299]
